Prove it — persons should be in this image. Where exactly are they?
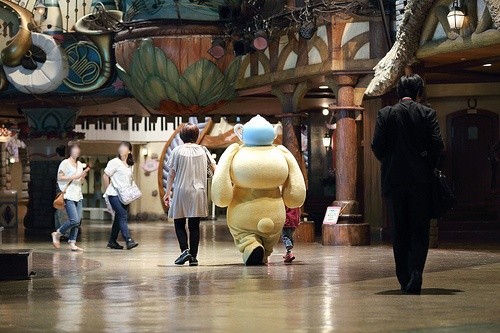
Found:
[51,142,87,251]
[56,145,72,242]
[282,206,300,262]
[165,123,216,266]
[372,73,444,294]
[103,141,138,250]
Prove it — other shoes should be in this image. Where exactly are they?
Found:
[401,285,420,295]
[243,241,268,266]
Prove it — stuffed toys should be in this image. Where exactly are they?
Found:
[211,115,306,266]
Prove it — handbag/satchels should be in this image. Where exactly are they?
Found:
[53,192,65,209]
[109,175,143,205]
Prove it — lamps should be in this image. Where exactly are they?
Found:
[447,0,465,30]
[299,22,318,40]
[252,30,268,52]
[207,39,227,60]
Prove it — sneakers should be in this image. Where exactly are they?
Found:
[69,243,78,251]
[175,250,198,266]
[283,252,295,262]
[126,237,138,250]
[107,241,124,249]
[51,232,60,248]
